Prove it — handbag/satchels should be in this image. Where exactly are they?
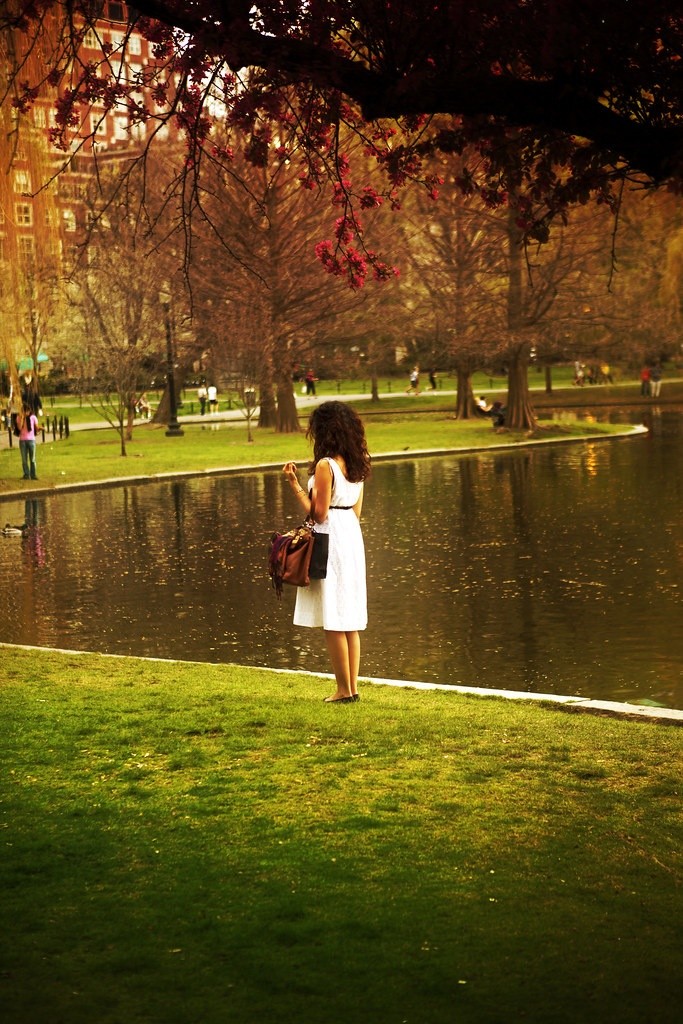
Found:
[267,487,330,602]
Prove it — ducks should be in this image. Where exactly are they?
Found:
[2,523,28,535]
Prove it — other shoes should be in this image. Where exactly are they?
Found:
[322,696,354,703]
[31,477,37,479]
[21,474,29,479]
[353,694,361,702]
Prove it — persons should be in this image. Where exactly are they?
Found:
[479,396,486,407]
[207,384,219,414]
[406,366,419,396]
[16,405,44,481]
[282,401,372,703]
[22,499,46,573]
[650,363,663,397]
[640,364,650,398]
[573,358,613,387]
[131,391,150,419]
[426,368,436,391]
[305,368,318,399]
[198,383,207,415]
